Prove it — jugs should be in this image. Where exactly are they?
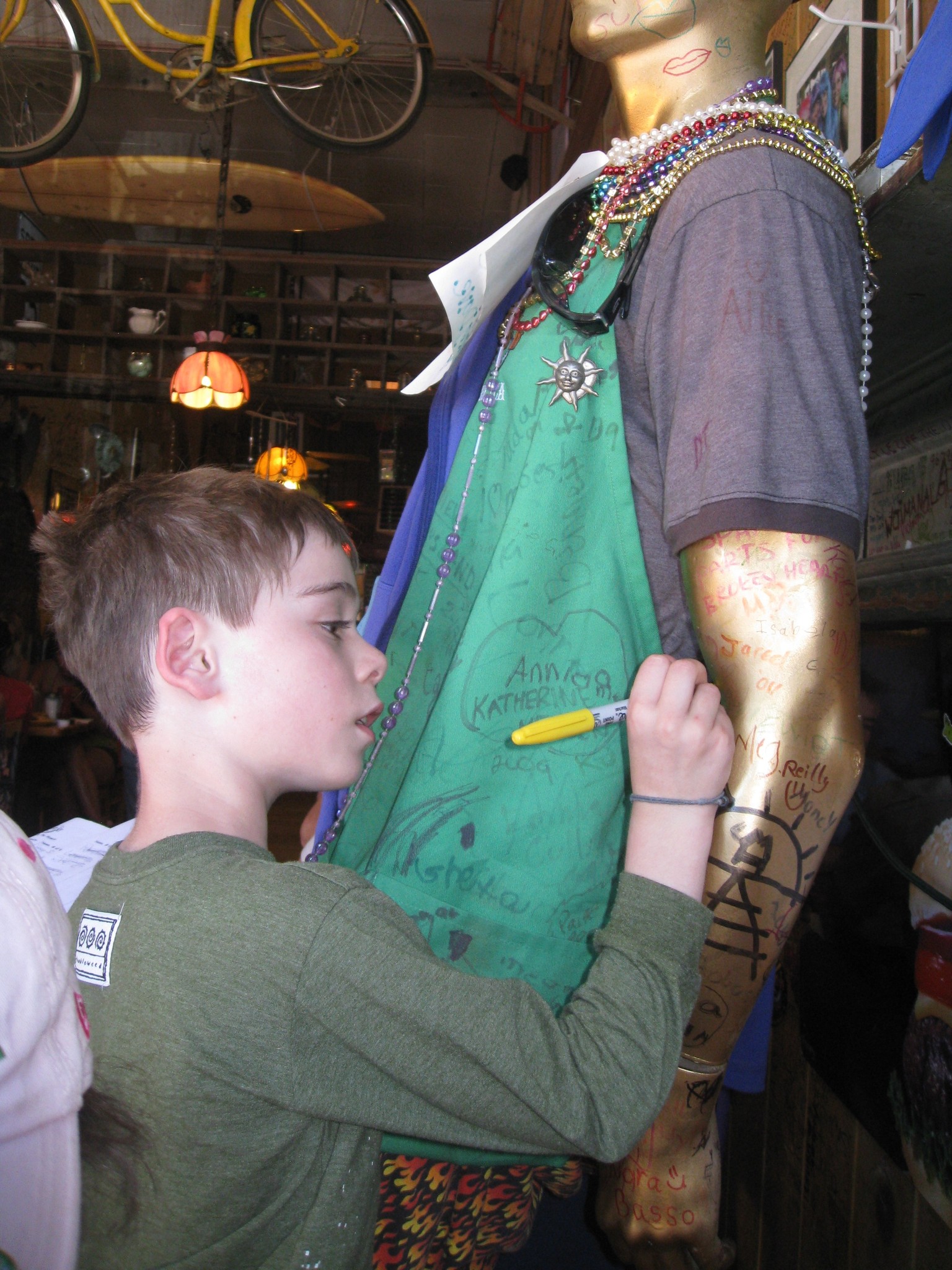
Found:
[129,307,167,334]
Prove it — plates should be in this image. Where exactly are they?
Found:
[14,319,50,327]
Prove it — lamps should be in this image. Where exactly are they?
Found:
[256,446,307,483]
[170,85,249,409]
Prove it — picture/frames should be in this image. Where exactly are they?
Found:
[784,0,877,166]
[765,40,783,104]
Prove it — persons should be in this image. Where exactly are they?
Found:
[301,0,867,1270]
[31,466,735,1270]
[0,808,93,1270]
[908,819,952,1199]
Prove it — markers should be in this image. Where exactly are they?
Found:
[512,700,628,745]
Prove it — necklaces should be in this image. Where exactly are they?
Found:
[305,78,881,863]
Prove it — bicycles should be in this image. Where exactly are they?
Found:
[0,0,436,170]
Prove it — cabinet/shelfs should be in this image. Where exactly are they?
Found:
[0,239,452,407]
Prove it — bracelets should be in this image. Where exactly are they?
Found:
[629,790,729,806]
[678,1052,727,1076]
